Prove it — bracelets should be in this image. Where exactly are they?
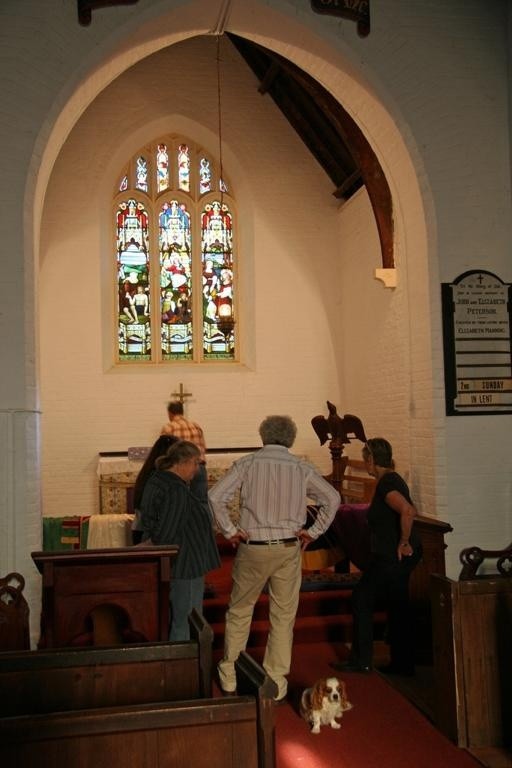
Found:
[399,542,408,547]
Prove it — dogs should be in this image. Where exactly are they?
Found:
[298,676,352,734]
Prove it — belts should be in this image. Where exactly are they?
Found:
[239,537,299,546]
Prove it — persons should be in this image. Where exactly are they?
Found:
[330,438,424,676]
[160,400,207,513]
[134,432,179,545]
[133,440,224,642]
[207,415,343,701]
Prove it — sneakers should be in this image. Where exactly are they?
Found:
[220,687,236,697]
[272,695,288,706]
[336,661,373,672]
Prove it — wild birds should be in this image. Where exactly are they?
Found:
[310,399,368,446]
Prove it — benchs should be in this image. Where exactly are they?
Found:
[428,572,512,748]
